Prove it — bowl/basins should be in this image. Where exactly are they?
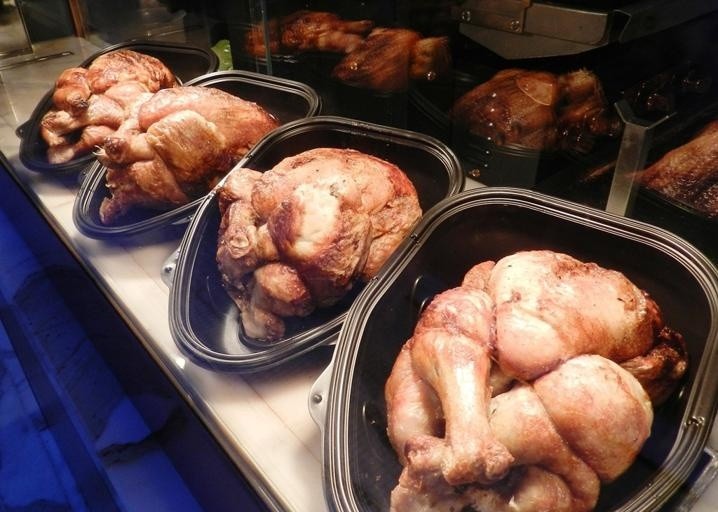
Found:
[161,103,463,377]
[69,71,324,237]
[306,177,718,508]
[16,32,186,173]
